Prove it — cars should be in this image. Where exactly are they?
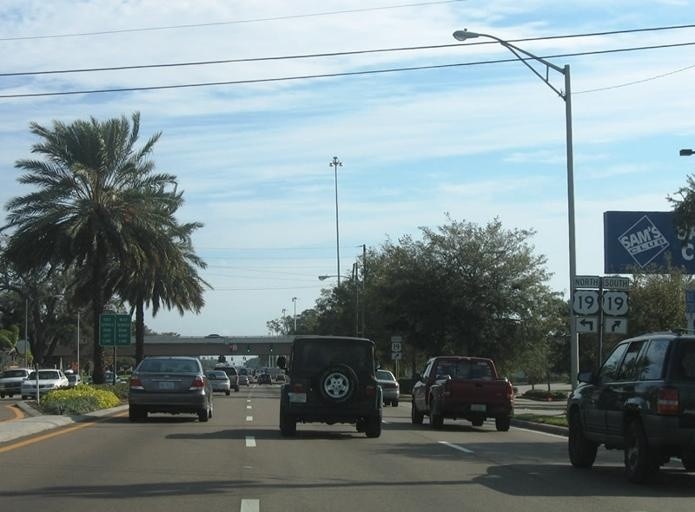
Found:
[1,370,29,398]
[214,366,240,392]
[129,356,215,422]
[66,373,82,387]
[237,367,287,386]
[21,369,69,400]
[205,334,225,338]
[104,372,122,384]
[205,371,230,395]
[375,368,401,408]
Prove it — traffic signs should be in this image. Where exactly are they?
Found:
[98,310,115,347]
[115,313,131,348]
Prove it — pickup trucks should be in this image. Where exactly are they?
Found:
[410,356,514,433]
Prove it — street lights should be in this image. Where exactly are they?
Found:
[451,26,581,390]
[318,274,360,336]
[24,294,65,370]
[329,153,342,290]
[281,306,286,332]
[292,296,297,332]
[678,148,695,156]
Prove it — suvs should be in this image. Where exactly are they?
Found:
[563,328,695,484]
[273,333,384,441]
